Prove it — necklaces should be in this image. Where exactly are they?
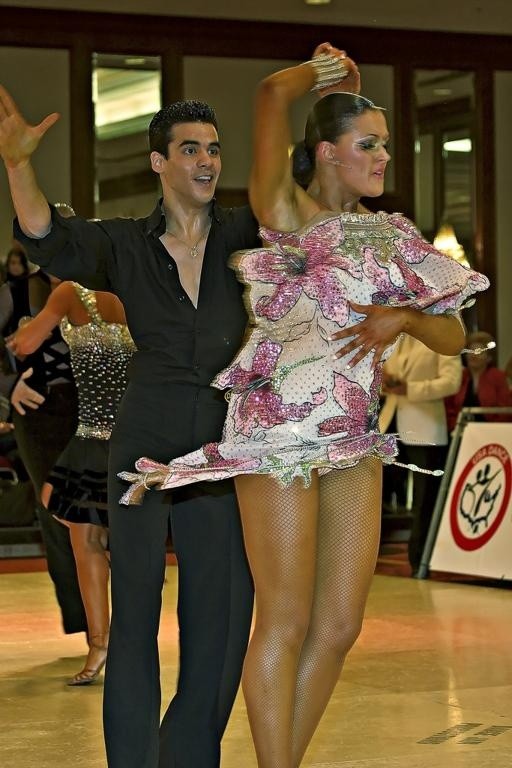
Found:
[163,226,210,259]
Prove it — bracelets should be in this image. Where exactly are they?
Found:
[304,55,348,91]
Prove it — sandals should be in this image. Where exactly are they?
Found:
[72,634,128,683]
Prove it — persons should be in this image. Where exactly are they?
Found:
[1,202,87,637]
[0,250,28,280]
[0,418,26,480]
[122,52,469,765]
[6,216,139,689]
[446,333,512,432]
[1,43,363,768]
[376,333,462,578]
